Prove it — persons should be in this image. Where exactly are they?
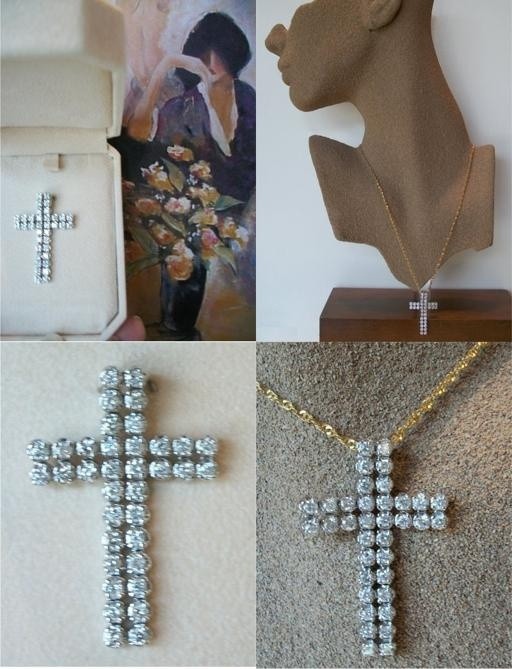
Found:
[262,0,498,287]
[122,12,255,342]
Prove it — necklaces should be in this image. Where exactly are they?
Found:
[357,143,477,339]
[256,341,495,661]
